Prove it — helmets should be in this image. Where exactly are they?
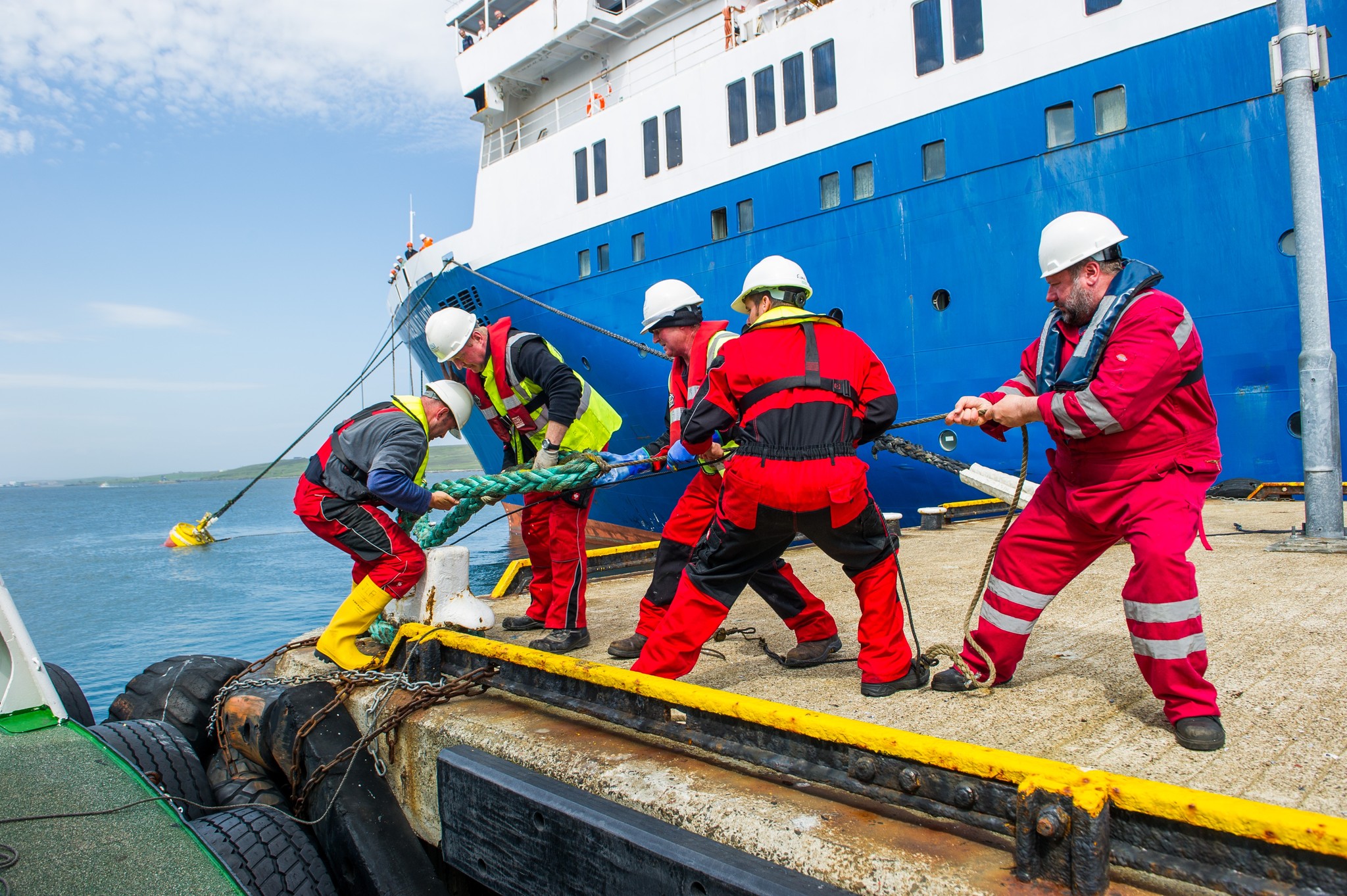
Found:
[730,255,813,313]
[422,307,477,363]
[1037,210,1128,278]
[639,279,704,334]
[425,379,474,440]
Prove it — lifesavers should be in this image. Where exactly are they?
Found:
[585,93,604,117]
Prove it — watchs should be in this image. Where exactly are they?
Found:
[541,438,561,451]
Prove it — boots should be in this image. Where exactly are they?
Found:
[312,573,393,672]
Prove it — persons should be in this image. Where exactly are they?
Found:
[628,251,933,697]
[386,232,433,285]
[426,307,624,655]
[580,279,842,669]
[494,9,510,29]
[458,30,474,54]
[476,19,493,41]
[931,210,1229,752]
[293,379,474,673]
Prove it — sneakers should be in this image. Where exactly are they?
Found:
[861,658,930,697]
[786,633,842,662]
[931,668,978,692]
[1174,714,1227,751]
[502,615,545,630]
[528,626,591,654]
[607,633,648,658]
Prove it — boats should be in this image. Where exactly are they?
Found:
[379,1,1347,565]
